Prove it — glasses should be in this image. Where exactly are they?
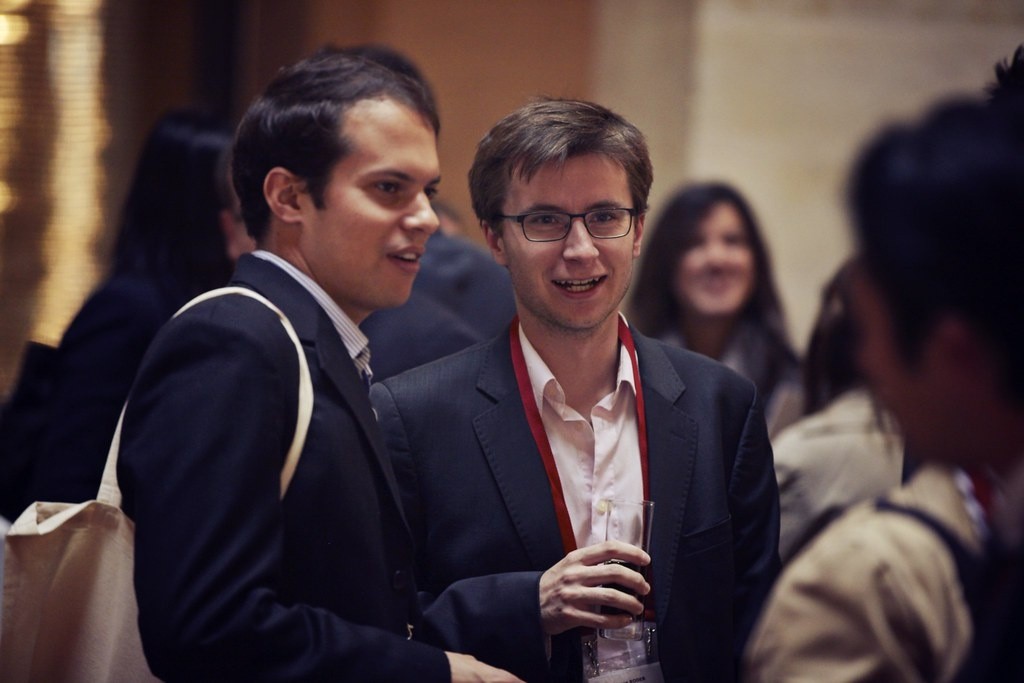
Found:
[502,207,638,241]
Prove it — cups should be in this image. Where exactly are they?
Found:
[598,499,655,640]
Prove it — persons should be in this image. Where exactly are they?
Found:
[58,43,519,493]
[368,97,779,683]
[767,45,1024,683]
[626,180,800,406]
[118,54,521,683]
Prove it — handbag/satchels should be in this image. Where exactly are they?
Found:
[0,286,314,683]
